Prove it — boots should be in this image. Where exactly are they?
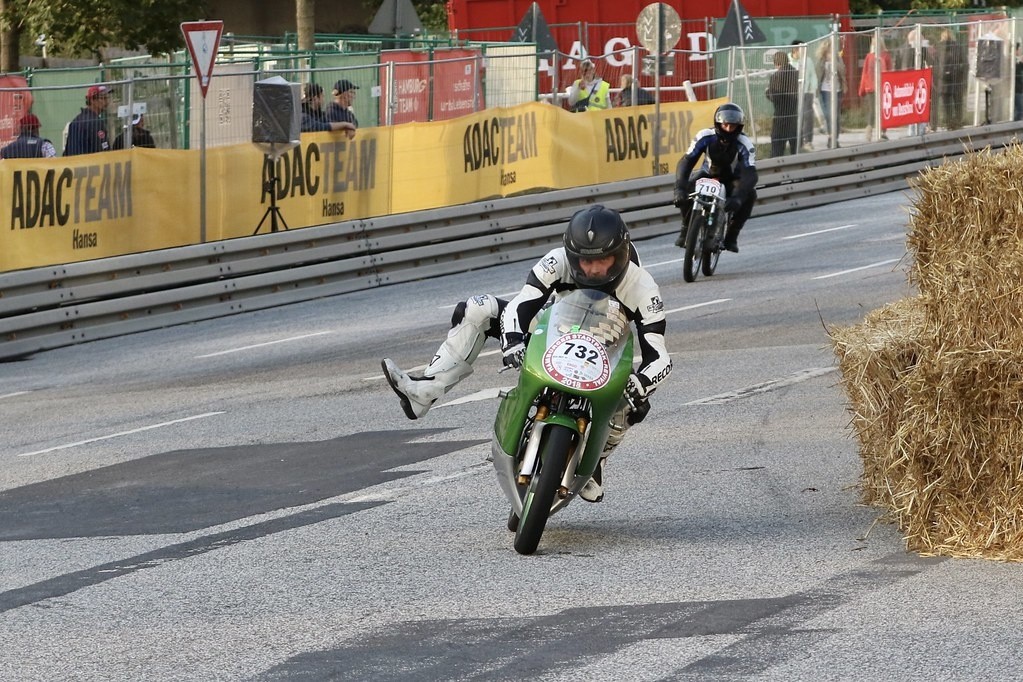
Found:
[676,219,689,246]
[382,341,475,420]
[723,220,742,252]
[580,421,626,503]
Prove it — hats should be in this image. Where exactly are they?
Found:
[86,84,112,97]
[20,115,41,128]
[302,84,323,103]
[333,80,360,95]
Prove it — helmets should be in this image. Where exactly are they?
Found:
[713,104,745,141]
[563,207,631,292]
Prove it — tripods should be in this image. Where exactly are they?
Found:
[253,142,287,235]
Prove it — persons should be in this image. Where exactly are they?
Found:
[1014,42,1023,95]
[382,204,672,503]
[765,51,798,158]
[858,36,893,141]
[112,114,156,150]
[300,83,356,141]
[0,114,57,159]
[612,74,656,108]
[62,86,114,157]
[931,30,969,131]
[673,103,758,253]
[814,38,848,149]
[892,31,940,137]
[326,79,360,129]
[568,61,612,113]
[788,40,817,152]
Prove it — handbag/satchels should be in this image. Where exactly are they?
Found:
[571,97,589,112]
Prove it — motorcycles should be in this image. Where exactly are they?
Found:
[486,286,638,555]
[680,174,732,283]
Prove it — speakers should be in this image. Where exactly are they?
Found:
[252,82,302,144]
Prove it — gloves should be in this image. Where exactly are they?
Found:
[673,189,687,207]
[624,372,657,410]
[725,192,747,212]
[500,332,532,368]
